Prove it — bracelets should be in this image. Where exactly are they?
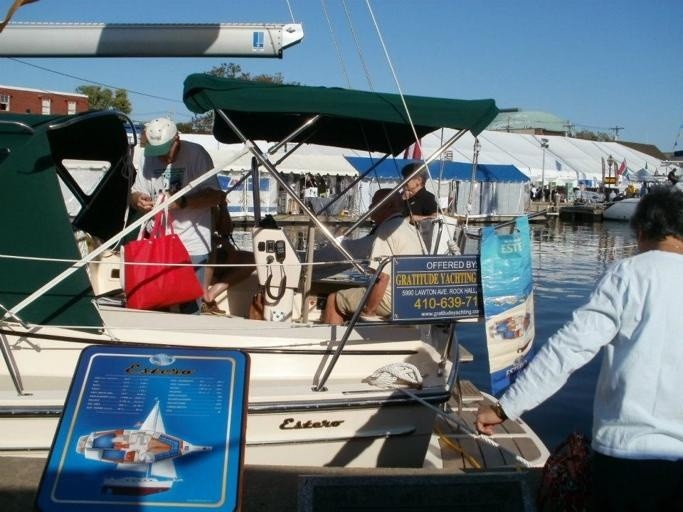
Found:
[179,196,187,209]
[494,404,507,420]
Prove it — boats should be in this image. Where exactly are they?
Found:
[0,6,551,507]
[604,175,683,223]
[73,430,213,468]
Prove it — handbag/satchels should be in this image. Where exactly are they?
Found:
[123,234,205,310]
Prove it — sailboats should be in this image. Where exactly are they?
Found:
[99,398,183,498]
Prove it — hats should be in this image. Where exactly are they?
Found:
[144,117,178,158]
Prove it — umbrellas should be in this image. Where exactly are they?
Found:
[627,168,659,184]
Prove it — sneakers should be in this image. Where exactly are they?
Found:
[201,299,226,316]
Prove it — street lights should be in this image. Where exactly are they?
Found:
[539,138,548,201]
[606,155,614,202]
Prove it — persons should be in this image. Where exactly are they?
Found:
[323,188,425,325]
[201,189,256,315]
[399,162,439,223]
[668,167,682,184]
[640,182,647,197]
[474,184,683,512]
[129,116,225,309]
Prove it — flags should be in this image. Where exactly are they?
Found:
[617,157,629,181]
